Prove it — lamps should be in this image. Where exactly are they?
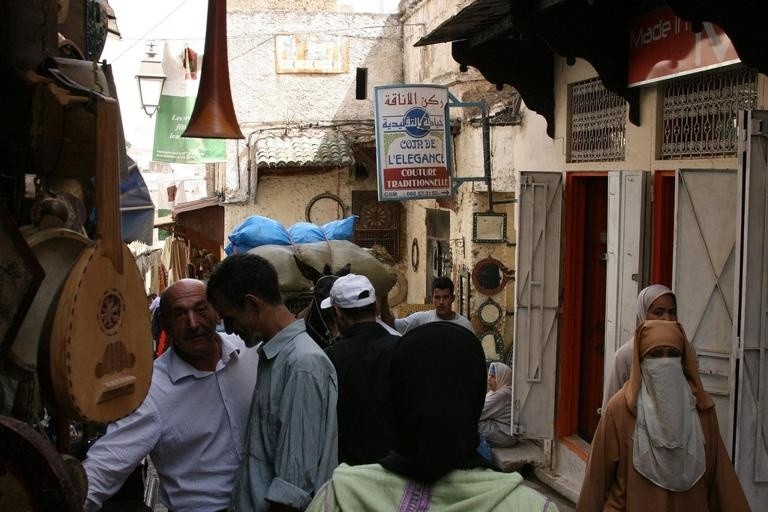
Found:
[134,39,167,120]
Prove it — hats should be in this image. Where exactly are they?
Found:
[319,273,377,310]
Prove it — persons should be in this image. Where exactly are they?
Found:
[79,276,263,512]
[205,253,341,511]
[377,275,477,339]
[574,318,751,511]
[477,359,520,469]
[600,283,680,411]
[319,273,402,465]
[146,293,160,314]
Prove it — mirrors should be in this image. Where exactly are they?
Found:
[411,238,418,272]
[473,210,507,243]
[472,256,515,295]
[477,328,505,366]
[456,267,470,321]
[478,297,503,328]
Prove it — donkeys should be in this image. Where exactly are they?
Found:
[292,252,353,347]
